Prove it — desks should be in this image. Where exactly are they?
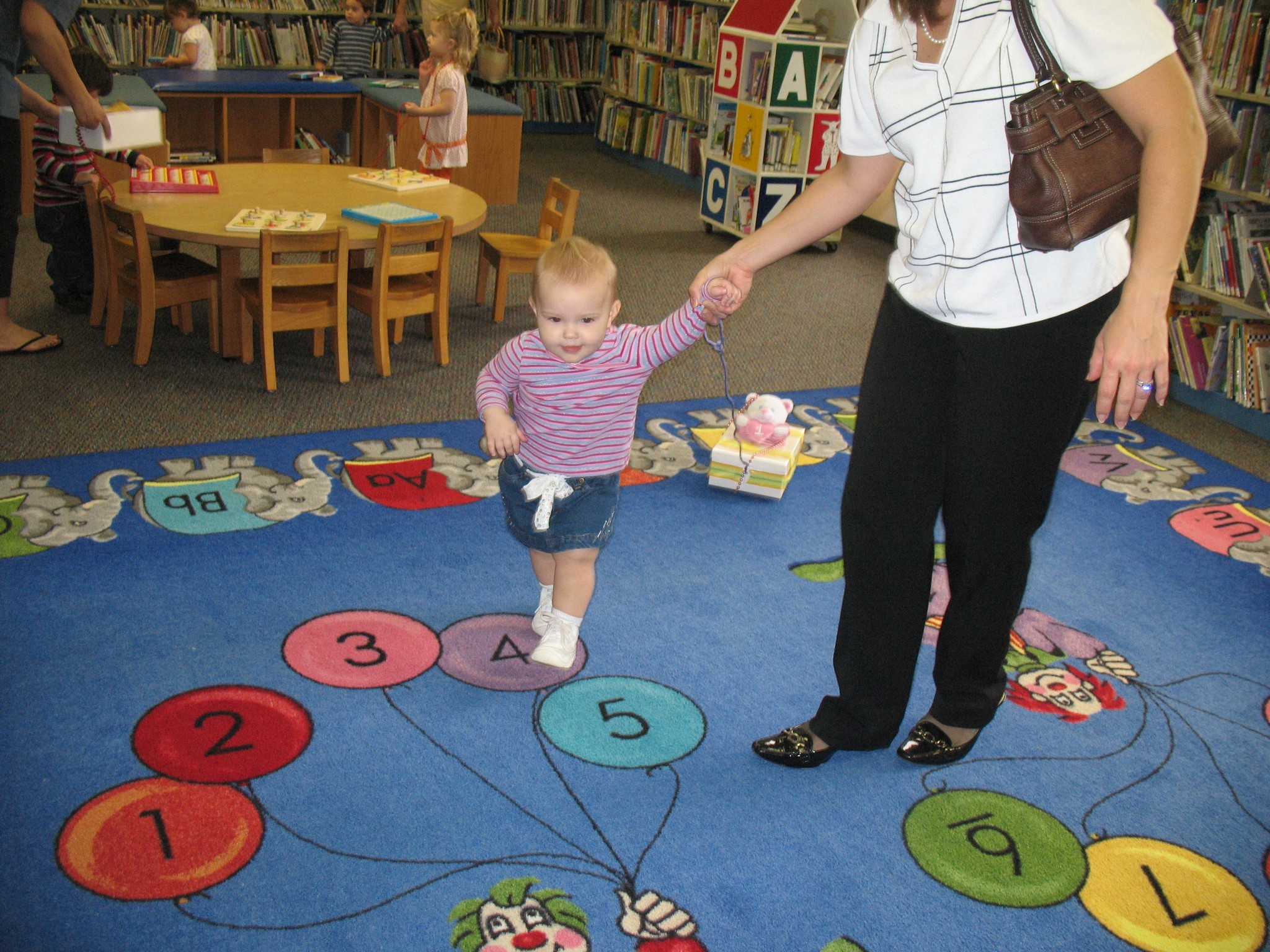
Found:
[103,163,489,360]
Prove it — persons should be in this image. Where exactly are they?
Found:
[32,43,154,314]
[161,0,217,71]
[313,0,397,71]
[393,0,471,54]
[687,0,1208,767]
[476,238,740,670]
[405,6,480,181]
[0,0,111,354]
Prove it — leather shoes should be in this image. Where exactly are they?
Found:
[751,723,838,767]
[897,692,1007,767]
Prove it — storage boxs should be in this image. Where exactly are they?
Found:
[708,420,806,501]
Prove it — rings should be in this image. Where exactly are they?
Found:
[1136,379,1153,391]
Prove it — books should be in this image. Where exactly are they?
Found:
[15,0,848,244]
[1159,0,1269,414]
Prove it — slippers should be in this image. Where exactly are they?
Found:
[0,327,64,354]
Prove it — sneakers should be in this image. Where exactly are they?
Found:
[530,609,579,668]
[530,585,554,635]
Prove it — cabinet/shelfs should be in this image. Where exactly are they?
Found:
[139,66,360,167]
[699,0,861,254]
[1159,87,1270,445]
[352,76,522,207]
[591,0,731,193]
[18,0,610,136]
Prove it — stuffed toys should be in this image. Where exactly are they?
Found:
[735,392,794,448]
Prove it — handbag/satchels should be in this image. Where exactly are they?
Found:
[1000,0,1245,252]
[476,25,508,84]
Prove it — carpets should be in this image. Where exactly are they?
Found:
[0,375,1270,952]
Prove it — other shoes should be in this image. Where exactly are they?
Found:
[54,293,92,312]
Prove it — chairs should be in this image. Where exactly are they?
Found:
[79,148,578,392]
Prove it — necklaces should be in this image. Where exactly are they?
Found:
[919,12,947,46]
